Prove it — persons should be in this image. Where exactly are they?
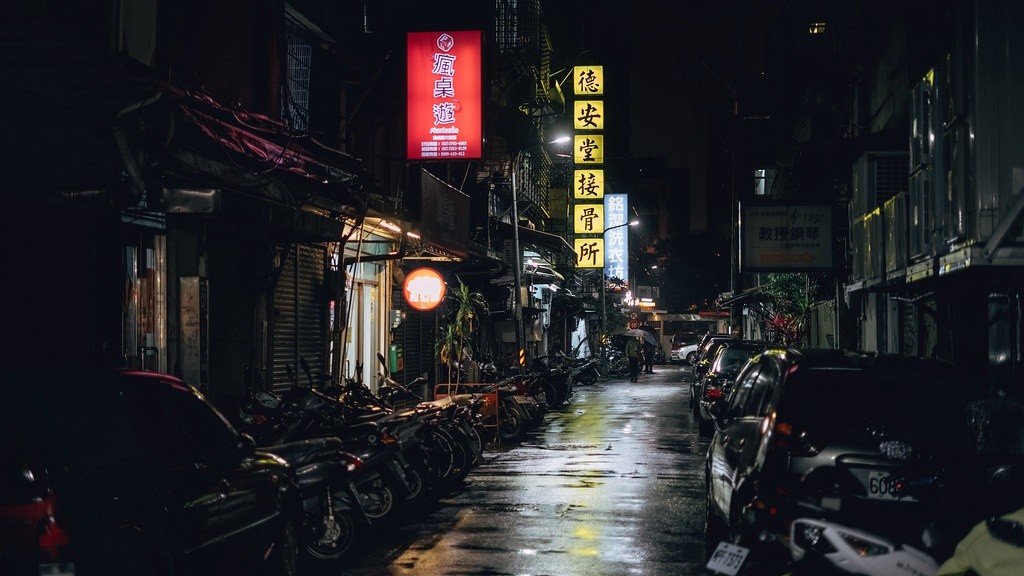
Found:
[625,337,639,382]
[643,341,653,371]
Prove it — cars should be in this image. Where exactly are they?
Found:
[639,342,666,366]
[688,337,739,407]
[0,360,307,576]
[703,343,990,566]
[695,341,786,439]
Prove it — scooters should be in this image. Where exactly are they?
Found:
[551,344,601,386]
[781,453,1023,576]
[531,353,574,410]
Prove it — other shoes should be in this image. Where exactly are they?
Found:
[630,377,634,381]
[634,377,638,382]
[643,369,648,373]
[648,369,652,373]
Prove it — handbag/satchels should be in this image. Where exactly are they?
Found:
[637,346,642,356]
[647,349,653,356]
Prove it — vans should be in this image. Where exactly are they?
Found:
[669,331,725,367]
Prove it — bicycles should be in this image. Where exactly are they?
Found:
[594,348,632,376]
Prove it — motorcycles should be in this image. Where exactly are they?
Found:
[223,352,557,560]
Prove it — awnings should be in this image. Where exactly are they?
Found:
[124,66,364,200]
[497,218,577,261]
[719,285,796,336]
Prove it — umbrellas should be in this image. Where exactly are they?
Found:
[609,325,658,346]
